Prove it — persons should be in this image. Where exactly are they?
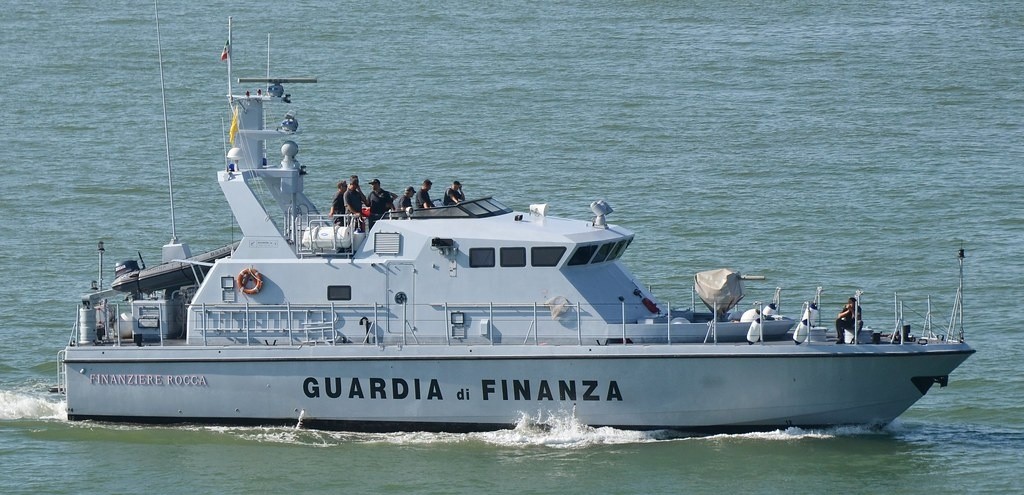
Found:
[836,298,863,344]
[328,180,347,227]
[415,179,435,208]
[444,181,465,206]
[397,187,416,210]
[344,175,366,227]
[364,179,395,231]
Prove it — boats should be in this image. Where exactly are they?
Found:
[54,0,974,435]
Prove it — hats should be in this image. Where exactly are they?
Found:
[368,179,380,183]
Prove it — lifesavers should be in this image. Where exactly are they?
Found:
[237,269,263,293]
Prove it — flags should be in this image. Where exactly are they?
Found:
[229,108,238,145]
[220,40,230,60]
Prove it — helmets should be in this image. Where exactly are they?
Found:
[405,186,416,193]
